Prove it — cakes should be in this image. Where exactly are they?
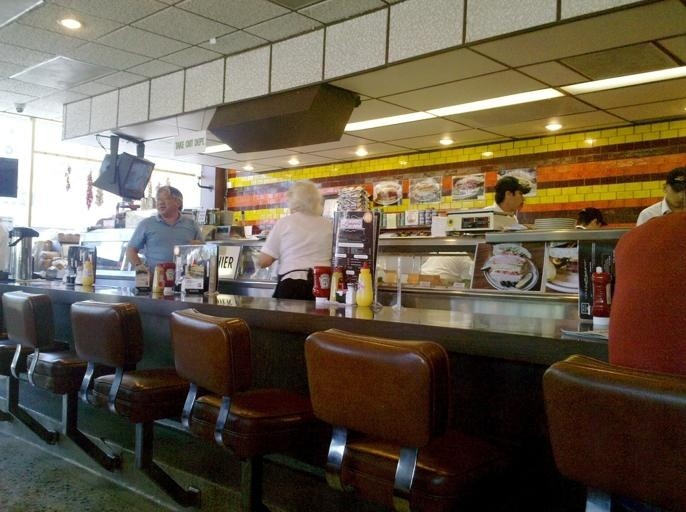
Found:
[488,252,526,283]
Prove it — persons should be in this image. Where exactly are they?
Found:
[126,186,206,292]
[607,213,685,374]
[576,207,607,229]
[258,182,334,301]
[636,166,685,226]
[481,175,532,230]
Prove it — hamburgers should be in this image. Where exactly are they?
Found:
[377,188,397,202]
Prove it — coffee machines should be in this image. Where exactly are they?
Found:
[6,227,42,282]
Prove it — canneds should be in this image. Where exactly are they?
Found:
[312,266,331,297]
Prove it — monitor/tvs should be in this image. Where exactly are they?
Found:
[0,157,19,199]
[92,151,155,200]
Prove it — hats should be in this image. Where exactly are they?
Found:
[495,176,531,195]
[666,167,686,183]
[578,206,608,225]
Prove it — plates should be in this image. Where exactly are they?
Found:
[481,243,540,292]
[498,169,536,196]
[372,179,403,207]
[547,241,594,295]
[452,175,484,201]
[252,234,272,241]
[534,218,576,233]
[410,177,441,204]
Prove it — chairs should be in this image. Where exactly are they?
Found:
[69,297,209,508]
[541,353,686,512]
[301,327,496,512]
[0,289,121,472]
[168,306,320,512]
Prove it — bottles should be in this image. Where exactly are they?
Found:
[135,267,151,295]
[164,262,176,291]
[82,255,94,288]
[416,209,440,225]
[65,273,79,287]
[344,282,358,307]
[330,267,346,304]
[356,262,373,307]
[151,264,167,295]
[313,265,332,306]
[590,272,613,324]
